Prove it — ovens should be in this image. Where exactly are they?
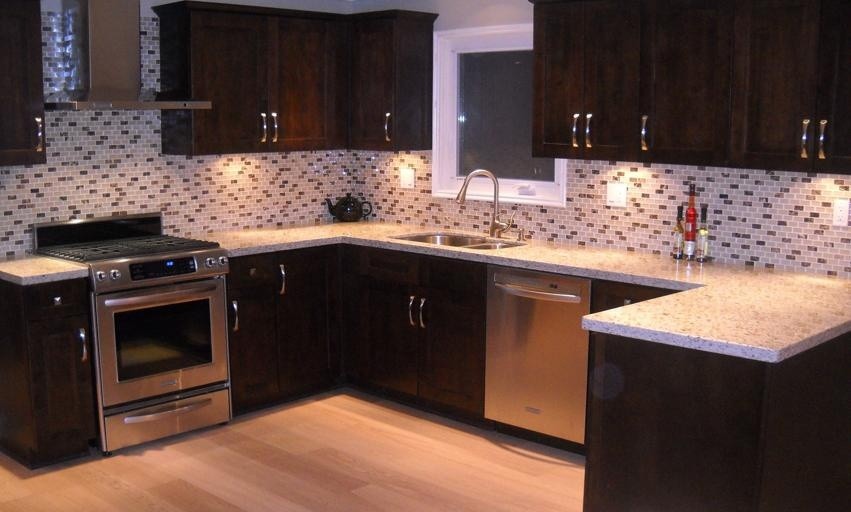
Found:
[92,250,232,454]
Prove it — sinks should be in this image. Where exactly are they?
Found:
[462,242,526,250]
[388,231,489,246]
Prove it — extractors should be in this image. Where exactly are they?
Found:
[41,1,215,114]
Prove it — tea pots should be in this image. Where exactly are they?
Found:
[323,192,374,223]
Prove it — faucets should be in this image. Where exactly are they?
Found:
[456,168,516,237]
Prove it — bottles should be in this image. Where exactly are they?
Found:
[673,205,683,261]
[696,204,709,264]
[683,182,696,262]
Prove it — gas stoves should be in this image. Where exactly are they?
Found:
[35,232,223,263]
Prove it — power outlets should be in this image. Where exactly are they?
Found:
[831,197,849,228]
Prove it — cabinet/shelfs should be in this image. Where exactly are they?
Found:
[276,245,336,401]
[527,0,639,163]
[152,1,349,157]
[348,9,439,152]
[582,279,850,511]
[0,280,98,471]
[335,243,487,413]
[1,0,47,167]
[727,0,849,175]
[639,2,728,168]
[225,252,281,410]
